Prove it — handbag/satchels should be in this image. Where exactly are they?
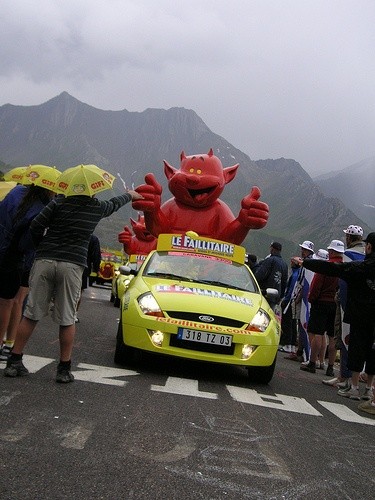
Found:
[0,246,24,299]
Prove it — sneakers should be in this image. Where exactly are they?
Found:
[4,358,30,376]
[361,386,374,399]
[360,399,375,414]
[55,370,74,383]
[337,384,361,400]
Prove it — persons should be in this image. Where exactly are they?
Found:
[246,224,375,415]
[118,214,158,255]
[0,164,145,385]
[117,148,269,255]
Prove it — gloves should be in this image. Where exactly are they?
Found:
[89,272,98,283]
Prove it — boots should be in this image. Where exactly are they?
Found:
[326,363,335,376]
[300,360,316,373]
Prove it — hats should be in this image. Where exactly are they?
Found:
[327,239,345,253]
[291,256,304,266]
[271,242,281,252]
[298,241,315,254]
[312,249,329,261]
[362,232,375,244]
[343,225,363,236]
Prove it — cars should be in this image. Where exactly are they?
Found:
[87,230,283,384]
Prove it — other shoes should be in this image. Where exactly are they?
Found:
[302,360,324,369]
[323,377,343,386]
[284,353,302,362]
[337,378,352,388]
[0,345,14,370]
[359,373,367,383]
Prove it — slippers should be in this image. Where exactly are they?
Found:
[278,347,296,354]
